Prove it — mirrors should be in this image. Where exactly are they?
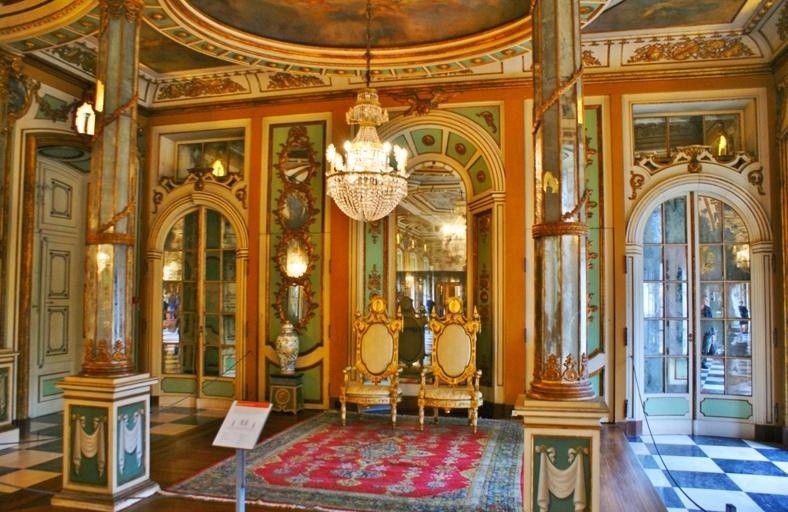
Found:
[388,153,473,386]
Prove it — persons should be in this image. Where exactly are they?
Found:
[701,294,715,369]
[738,300,749,334]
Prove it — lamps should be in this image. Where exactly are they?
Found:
[324,0,408,222]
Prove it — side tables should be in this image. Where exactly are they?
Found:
[268,372,305,415]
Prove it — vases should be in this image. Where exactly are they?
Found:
[276,321,300,374]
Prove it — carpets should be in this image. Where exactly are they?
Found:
[398,364,465,385]
[156,409,524,512]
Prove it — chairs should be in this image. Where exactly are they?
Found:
[398,296,426,369]
[417,297,485,425]
[339,296,405,422]
